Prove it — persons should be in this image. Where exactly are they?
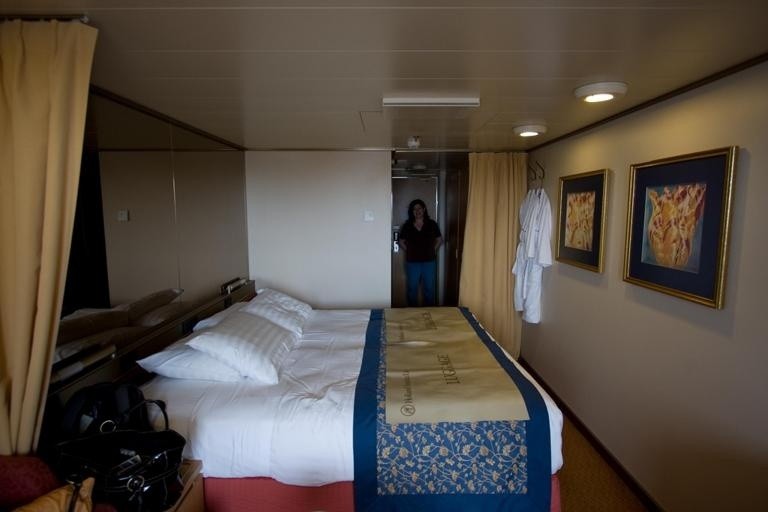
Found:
[395,199,443,307]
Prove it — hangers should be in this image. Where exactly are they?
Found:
[520,175,550,203]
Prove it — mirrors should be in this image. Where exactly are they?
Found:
[50,86,249,397]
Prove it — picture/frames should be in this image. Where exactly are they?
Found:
[554,145,738,310]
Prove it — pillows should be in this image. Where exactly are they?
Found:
[137,286,314,386]
[53,290,198,383]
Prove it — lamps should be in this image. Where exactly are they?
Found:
[512,82,628,137]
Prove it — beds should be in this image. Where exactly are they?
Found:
[50,281,565,512]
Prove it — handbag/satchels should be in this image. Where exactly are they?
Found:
[36,380,187,512]
